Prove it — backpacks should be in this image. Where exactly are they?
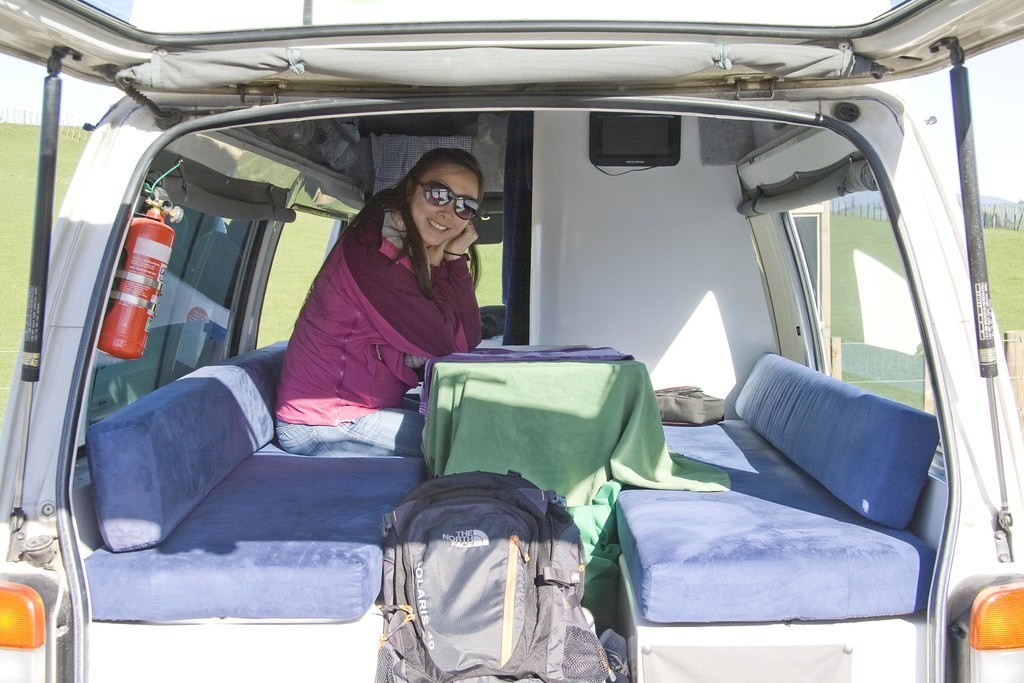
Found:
[377,470,612,683]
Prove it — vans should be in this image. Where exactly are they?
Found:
[2,0,1022,683]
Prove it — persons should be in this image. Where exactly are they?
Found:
[274,147,484,459]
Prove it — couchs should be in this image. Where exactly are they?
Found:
[612,349,938,683]
[73,338,429,683]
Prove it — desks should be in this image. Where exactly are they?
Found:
[417,342,653,507]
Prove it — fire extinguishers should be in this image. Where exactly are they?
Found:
[94,183,185,360]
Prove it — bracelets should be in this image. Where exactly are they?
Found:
[443,250,464,256]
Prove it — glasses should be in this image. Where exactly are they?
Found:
[414,178,479,221]
